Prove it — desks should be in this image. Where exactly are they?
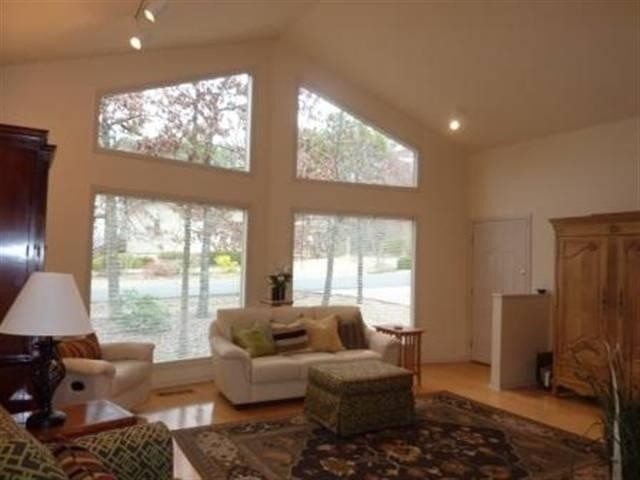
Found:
[20,397,139,465]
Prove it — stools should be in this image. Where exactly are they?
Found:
[376,318,423,384]
[300,360,413,430]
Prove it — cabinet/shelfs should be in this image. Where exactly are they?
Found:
[547,209,640,401]
[1,123,60,365]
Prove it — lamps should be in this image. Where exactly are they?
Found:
[124,0,170,54]
[0,269,92,429]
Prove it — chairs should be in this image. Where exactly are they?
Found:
[51,329,157,413]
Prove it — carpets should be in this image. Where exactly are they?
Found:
[170,387,612,480]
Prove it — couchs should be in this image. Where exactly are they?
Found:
[208,305,400,420]
[0,405,173,480]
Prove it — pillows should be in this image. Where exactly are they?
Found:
[232,312,370,355]
[58,332,105,360]
